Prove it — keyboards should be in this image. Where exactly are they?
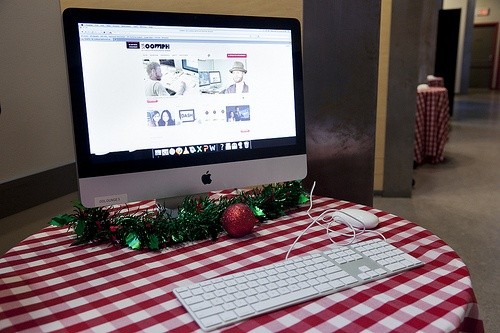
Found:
[173,238,426,332]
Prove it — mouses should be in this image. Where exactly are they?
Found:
[334,208,379,230]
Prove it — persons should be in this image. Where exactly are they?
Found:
[232,106,247,121]
[226,111,236,121]
[220,62,250,93]
[158,110,175,126]
[145,59,187,96]
[149,110,161,125]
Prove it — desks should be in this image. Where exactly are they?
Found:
[413,86,449,166]
[0,187,486,333]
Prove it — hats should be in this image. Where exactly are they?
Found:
[229,61,247,74]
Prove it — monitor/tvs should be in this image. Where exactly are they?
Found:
[61,8,308,209]
